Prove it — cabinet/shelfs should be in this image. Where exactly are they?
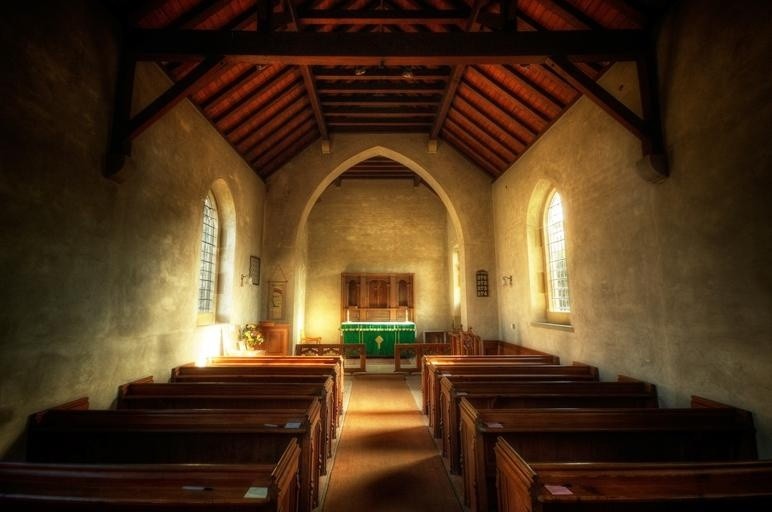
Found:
[340,271,416,324]
[255,323,290,356]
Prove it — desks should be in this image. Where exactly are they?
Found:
[0,353,349,512]
[339,321,417,359]
[418,351,767,510]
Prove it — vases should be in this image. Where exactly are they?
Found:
[245,339,254,350]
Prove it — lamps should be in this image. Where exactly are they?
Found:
[501,274,513,287]
[240,273,254,289]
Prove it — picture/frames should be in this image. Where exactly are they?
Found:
[248,255,261,286]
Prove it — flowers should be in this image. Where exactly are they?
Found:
[238,322,262,344]
